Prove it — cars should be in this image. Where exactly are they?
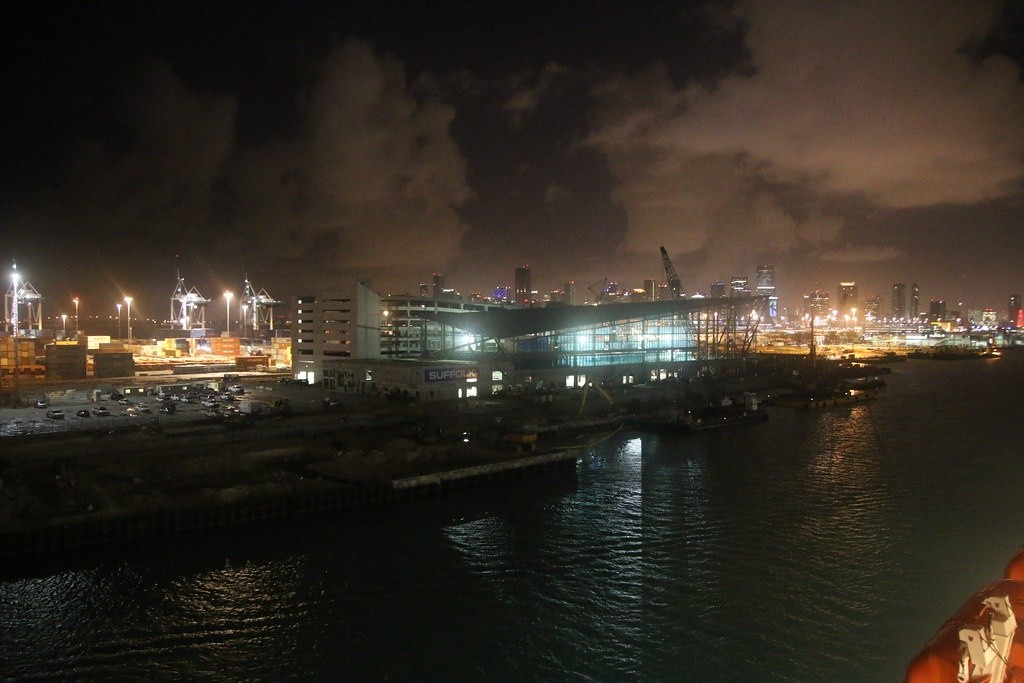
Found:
[33,394,225,421]
